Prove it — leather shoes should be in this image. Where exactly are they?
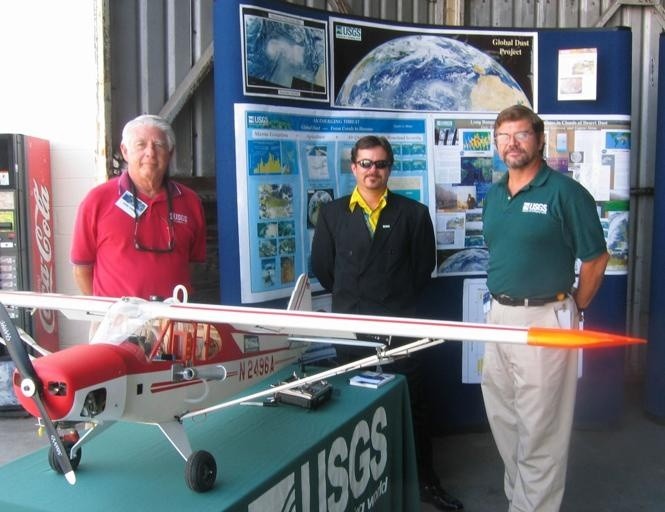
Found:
[420,480,464,512]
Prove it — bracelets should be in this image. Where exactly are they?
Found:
[578,307,585,313]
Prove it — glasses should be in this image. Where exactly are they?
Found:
[357,159,389,168]
[134,222,173,252]
[494,133,529,144]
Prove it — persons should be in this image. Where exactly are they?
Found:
[71,113,208,347]
[481,105,610,512]
[310,134,465,511]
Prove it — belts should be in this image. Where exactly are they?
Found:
[492,293,567,306]
